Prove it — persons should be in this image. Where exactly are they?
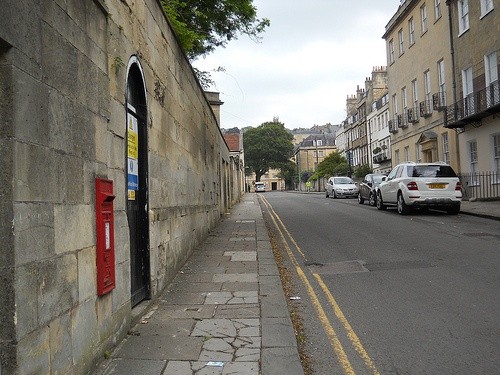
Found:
[305,179,312,192]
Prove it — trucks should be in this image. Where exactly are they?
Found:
[254,182,266,192]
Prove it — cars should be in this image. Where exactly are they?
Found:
[325,176,359,199]
[358,174,388,207]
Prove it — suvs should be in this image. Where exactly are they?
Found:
[375,160,463,215]
